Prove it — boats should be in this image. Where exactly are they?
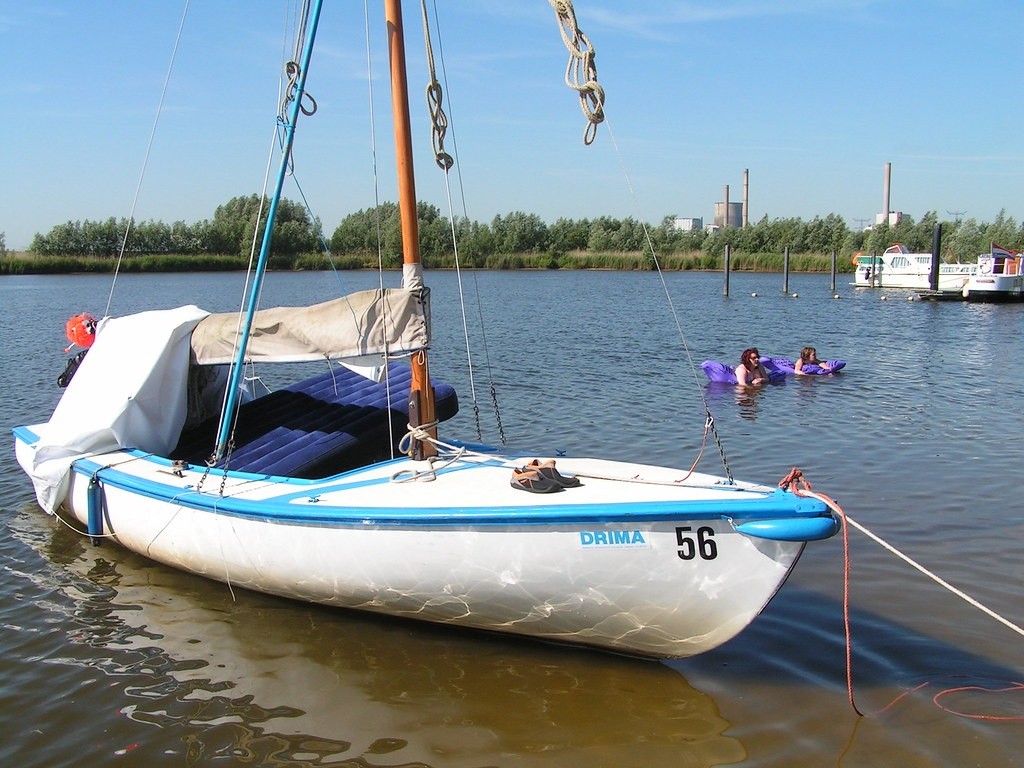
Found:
[847,243,1024,300]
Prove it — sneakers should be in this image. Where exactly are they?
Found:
[522,459,580,488]
[511,467,562,494]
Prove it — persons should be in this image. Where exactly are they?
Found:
[795,347,832,376]
[734,347,770,385]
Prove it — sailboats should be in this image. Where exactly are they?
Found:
[12,1,848,664]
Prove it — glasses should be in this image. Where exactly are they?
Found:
[750,356,760,361]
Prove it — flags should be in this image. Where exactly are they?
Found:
[992,242,1015,261]
